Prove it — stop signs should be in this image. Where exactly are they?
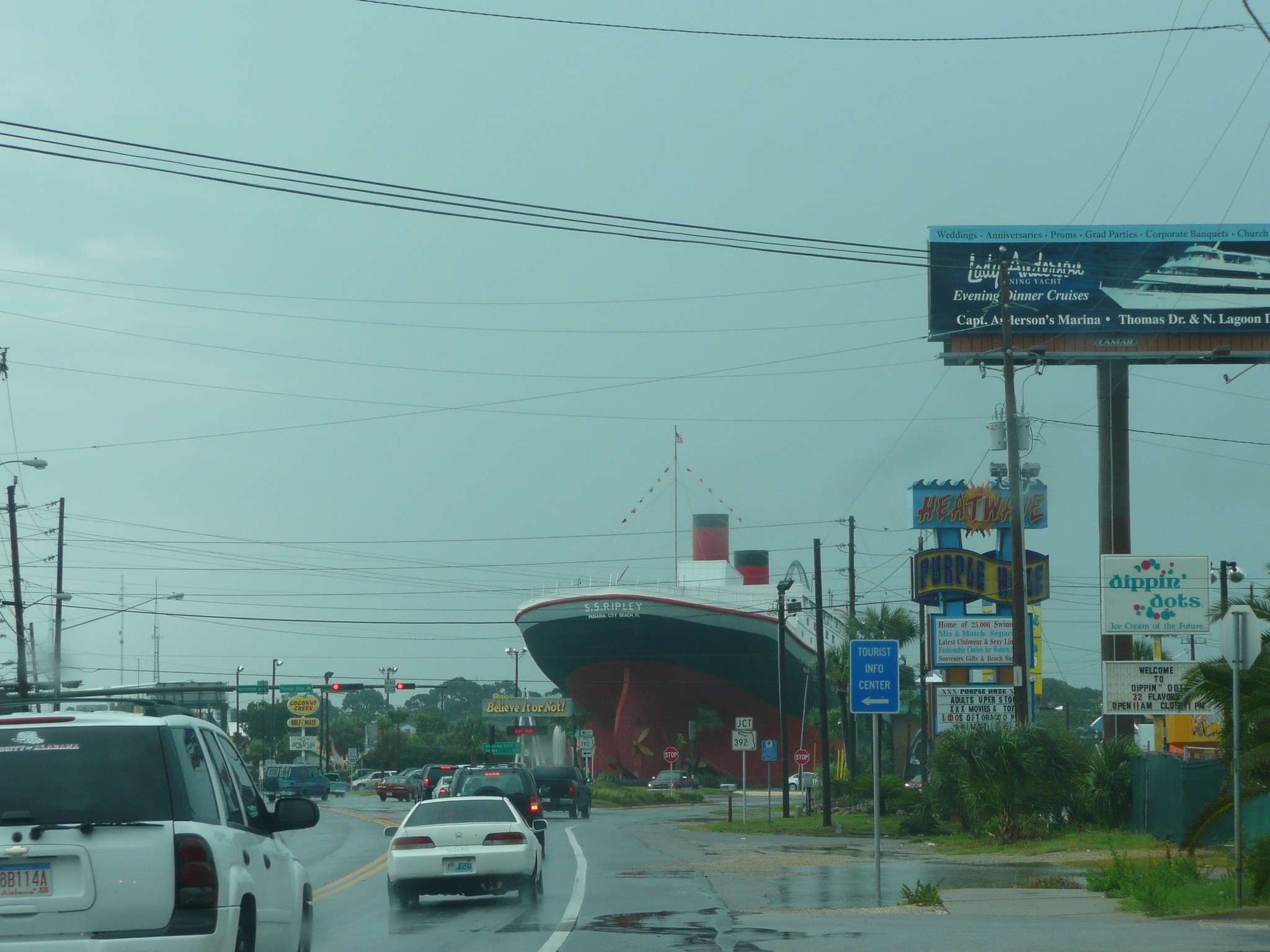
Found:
[794,749,810,765]
[515,426,873,790]
[664,747,678,763]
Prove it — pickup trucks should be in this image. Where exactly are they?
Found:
[648,770,702,790]
[323,773,346,797]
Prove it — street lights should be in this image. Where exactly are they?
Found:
[1211,560,1246,619]
[776,579,803,818]
[271,658,283,758]
[54,591,184,714]
[504,647,528,725]
[16,593,73,714]
[235,667,246,751]
[378,667,398,711]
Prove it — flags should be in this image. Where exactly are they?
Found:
[676,432,683,443]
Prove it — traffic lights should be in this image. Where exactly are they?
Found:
[397,683,416,690]
[331,683,364,691]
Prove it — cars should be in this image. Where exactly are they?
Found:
[384,796,548,906]
[378,776,417,801]
[788,772,822,790]
[398,767,421,789]
[431,776,452,800]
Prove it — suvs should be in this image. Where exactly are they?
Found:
[414,764,454,803]
[264,763,331,801]
[449,764,547,856]
[0,710,319,951]
[350,770,397,790]
